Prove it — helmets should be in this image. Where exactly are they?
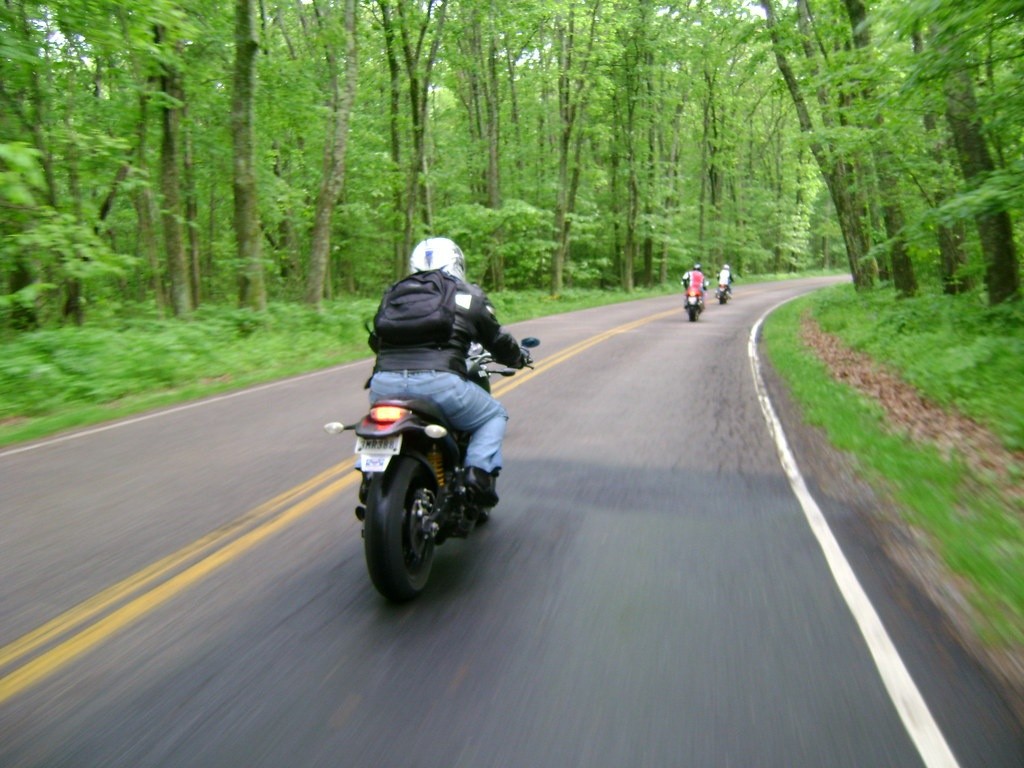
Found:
[694,264,701,270]
[410,238,466,281]
[723,265,729,270]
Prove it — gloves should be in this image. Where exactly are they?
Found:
[507,347,530,369]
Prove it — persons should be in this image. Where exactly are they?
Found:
[716,264,733,298]
[682,263,707,306]
[355,237,530,507]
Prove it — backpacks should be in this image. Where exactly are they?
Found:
[369,271,457,351]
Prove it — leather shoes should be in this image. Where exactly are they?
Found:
[464,466,499,506]
[359,472,374,505]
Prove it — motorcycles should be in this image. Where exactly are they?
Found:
[716,274,737,305]
[681,281,710,322]
[325,337,540,607]
